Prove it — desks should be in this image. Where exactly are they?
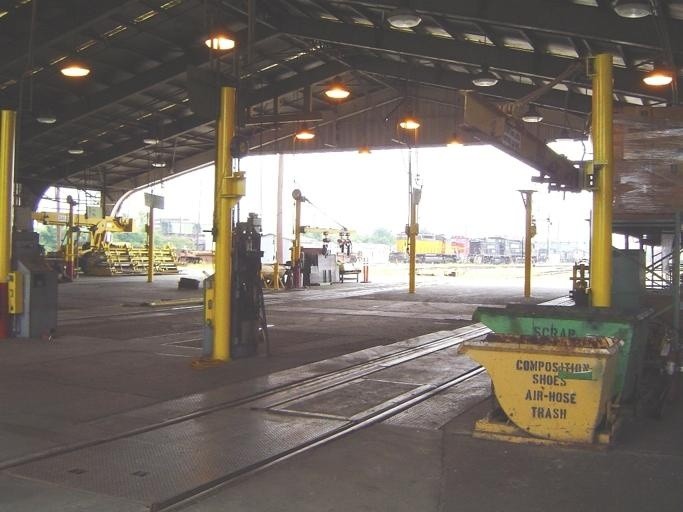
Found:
[338,270,361,284]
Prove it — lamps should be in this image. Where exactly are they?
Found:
[68,144,84,155]
[521,81,544,123]
[324,76,352,99]
[36,113,56,124]
[446,133,464,148]
[612,1,653,19]
[643,57,673,86]
[473,34,501,87]
[385,6,423,27]
[399,111,421,130]
[205,24,240,50]
[143,134,160,145]
[60,52,90,77]
[296,122,315,139]
[358,146,372,154]
[152,154,167,167]
